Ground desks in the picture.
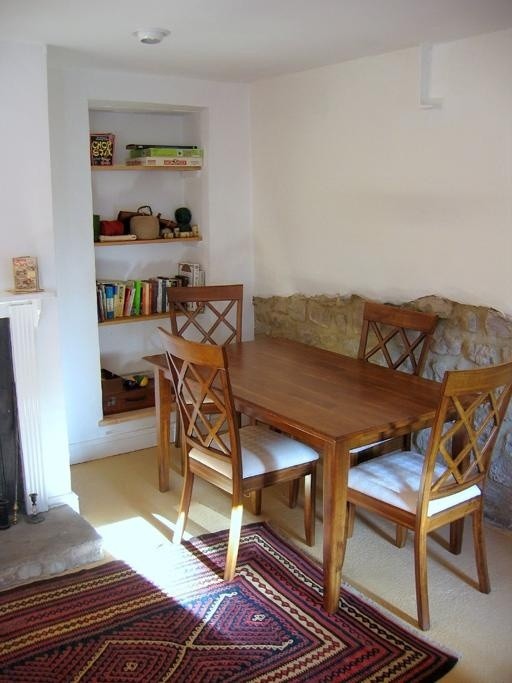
[142,335,482,616]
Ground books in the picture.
[96,263,206,323]
[98,234,137,243]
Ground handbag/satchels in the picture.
[118,205,176,229]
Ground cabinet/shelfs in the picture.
[95,166,202,427]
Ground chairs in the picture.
[344,361,511,630]
[356,302,439,462]
[159,327,319,584]
[167,286,243,448]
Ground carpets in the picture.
[2,521,459,681]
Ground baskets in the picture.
[103,377,169,416]
